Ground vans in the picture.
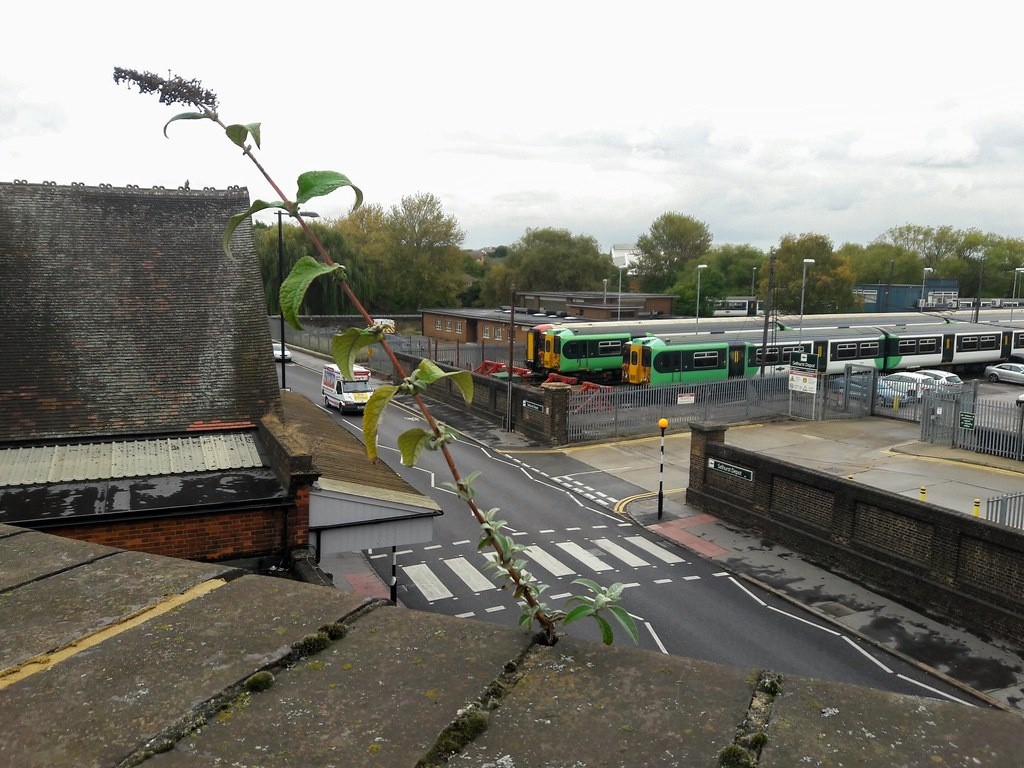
[321,364,374,415]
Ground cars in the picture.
[273,343,292,364]
[831,369,964,409]
[985,363,1024,386]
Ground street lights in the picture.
[695,264,708,336]
[920,267,933,312]
[1010,268,1024,324]
[273,209,321,388]
[603,279,608,305]
[798,258,816,353]
[618,266,623,321]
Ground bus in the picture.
[523,307,1024,403]
